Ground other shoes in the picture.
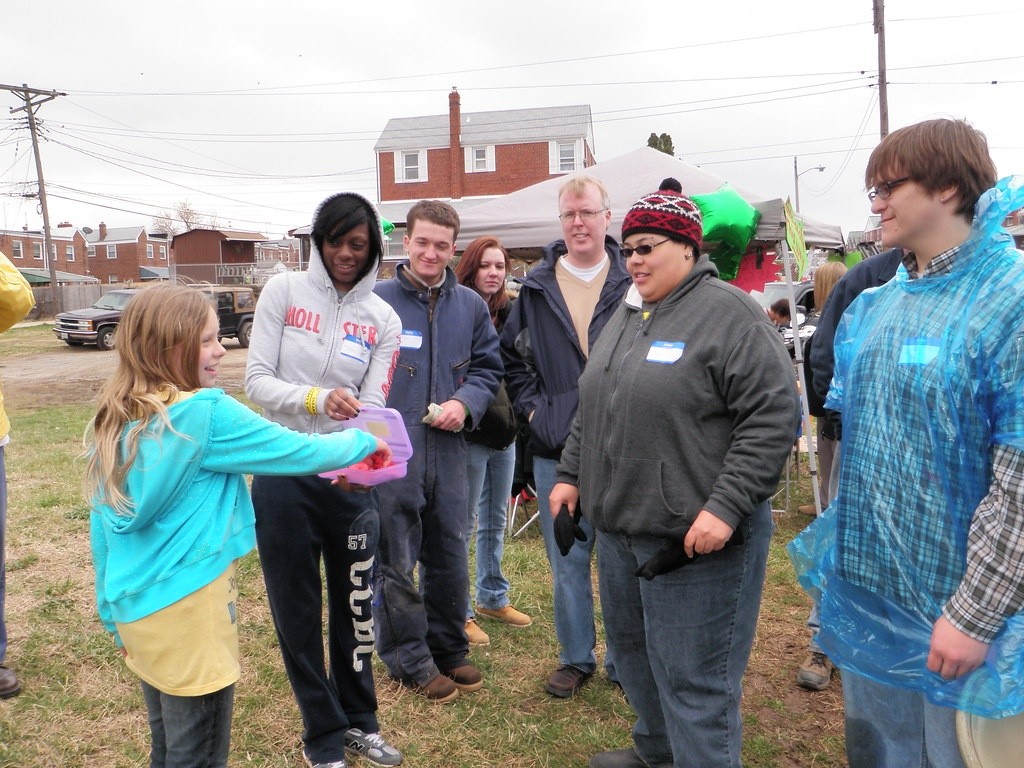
[798,505,817,514]
[1,662,22,697]
[442,665,484,691]
[590,749,645,767]
[406,673,459,702]
[545,665,588,697]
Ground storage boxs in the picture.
[315,412,419,486]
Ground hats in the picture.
[621,178,705,257]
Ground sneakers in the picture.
[463,617,491,647]
[475,604,532,628]
[302,748,347,768]
[344,727,403,767]
[797,651,835,689]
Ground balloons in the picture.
[690,180,762,280]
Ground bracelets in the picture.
[463,403,469,416]
[305,387,322,415]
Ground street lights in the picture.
[792,155,827,214]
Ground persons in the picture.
[0,251,36,699]
[786,118,1024,768]
[82,283,392,768]
[499,175,632,698]
[795,246,910,691]
[550,178,804,767]
[798,261,847,515]
[454,237,533,645]
[763,298,791,333]
[372,200,503,703]
[242,191,403,768]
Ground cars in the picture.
[50,288,146,351]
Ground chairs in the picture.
[770,396,803,513]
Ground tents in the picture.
[287,146,844,519]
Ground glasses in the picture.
[558,209,608,222]
[867,176,920,204]
[619,237,673,258]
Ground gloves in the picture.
[554,494,587,557]
[634,523,744,581]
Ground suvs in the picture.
[194,286,259,348]
